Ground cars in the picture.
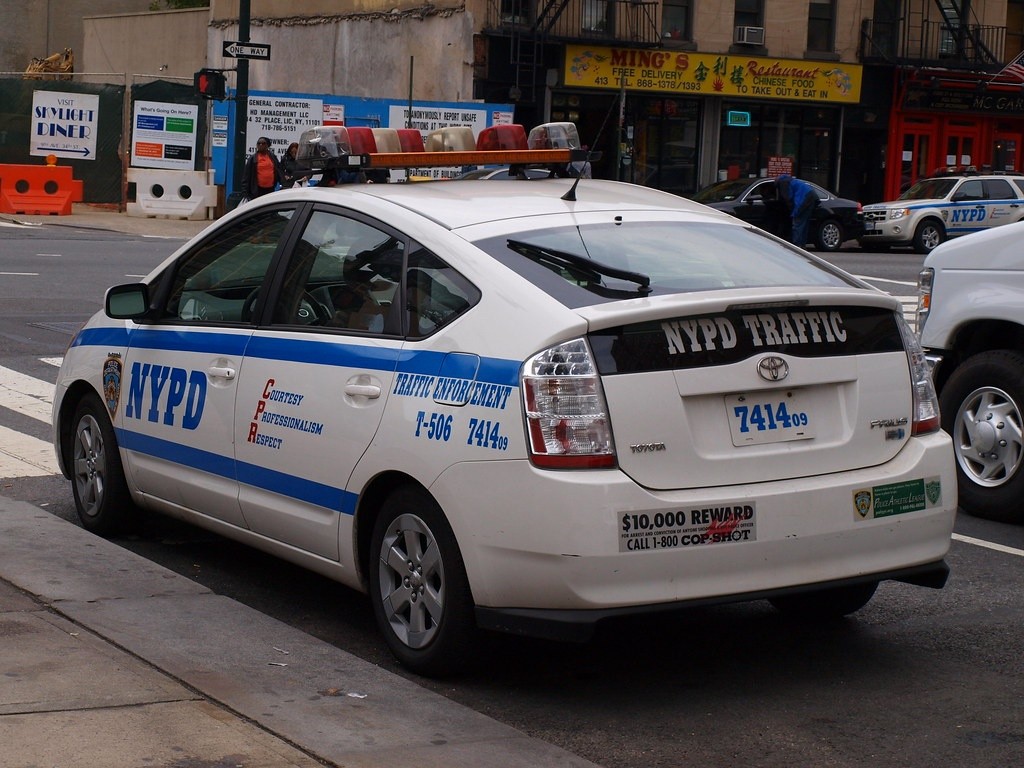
[52,121,960,678]
[691,175,865,251]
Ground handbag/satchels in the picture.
[568,162,582,179]
[236,198,248,208]
[292,181,301,189]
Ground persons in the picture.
[566,142,591,179]
[280,143,313,190]
[774,173,819,250]
[753,185,768,199]
[242,137,286,200]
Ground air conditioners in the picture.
[733,26,765,46]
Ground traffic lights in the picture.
[195,71,228,102]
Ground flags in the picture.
[1003,54,1024,81]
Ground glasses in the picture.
[257,142,267,145]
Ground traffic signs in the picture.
[221,39,272,60]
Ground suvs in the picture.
[916,218,1024,519]
[863,163,1024,254]
